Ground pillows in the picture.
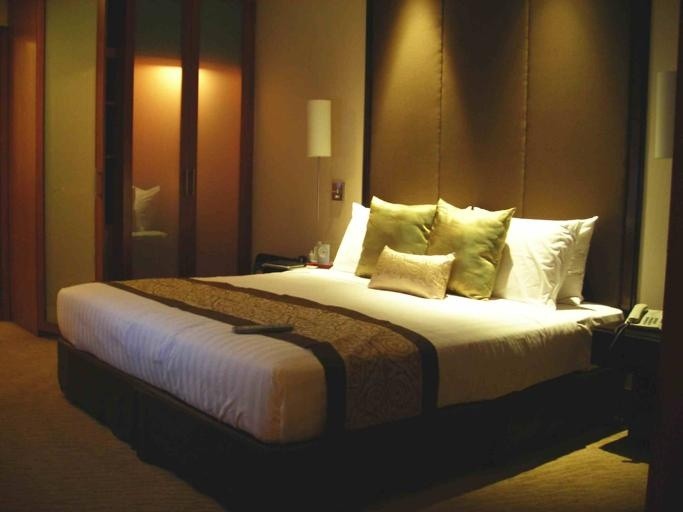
[332,195,601,304]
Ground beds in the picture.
[55,260,626,510]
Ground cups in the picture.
[318,240,330,265]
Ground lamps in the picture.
[307,99,332,252]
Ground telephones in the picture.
[625,304,663,330]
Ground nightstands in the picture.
[591,320,660,444]
[252,253,312,273]
